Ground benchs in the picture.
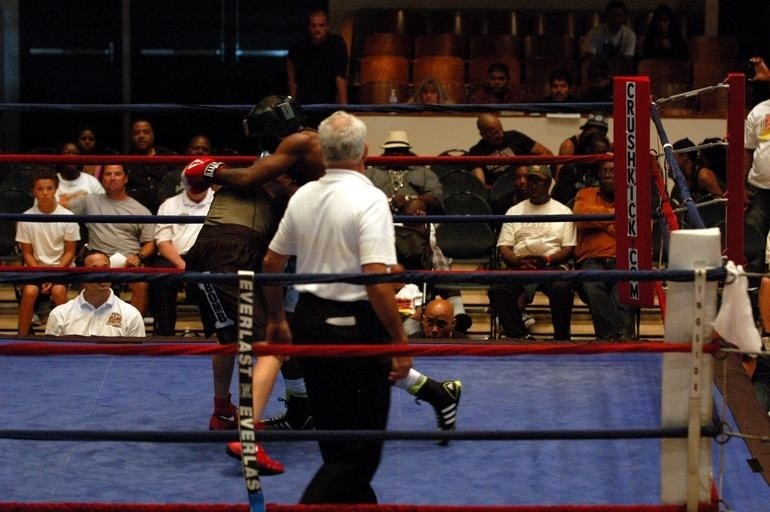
[1,134,733,344]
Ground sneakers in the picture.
[259,408,317,432]
[31,308,51,325]
[223,440,286,474]
[428,379,463,448]
[587,328,641,345]
[499,333,540,344]
[521,311,536,329]
[453,313,473,333]
[209,402,269,431]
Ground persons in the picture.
[13,2,770,351]
[185,175,293,476]
[182,91,462,447]
[265,109,414,504]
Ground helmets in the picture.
[242,95,308,155]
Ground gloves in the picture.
[179,158,226,195]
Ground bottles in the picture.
[389,88,399,115]
[183,326,193,337]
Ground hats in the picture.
[580,111,609,132]
[523,164,552,180]
[379,130,413,150]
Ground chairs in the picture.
[356,2,742,105]
